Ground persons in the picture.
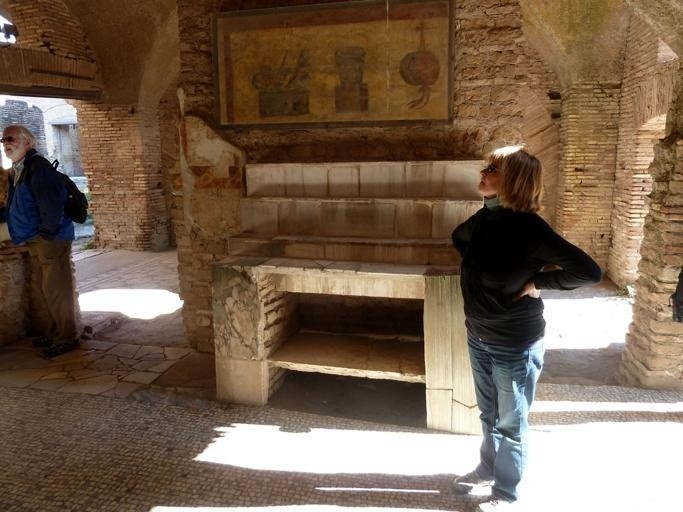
[0,123,79,360]
[452,145,604,512]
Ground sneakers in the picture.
[476,495,517,512]
[453,469,495,492]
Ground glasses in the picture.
[0,136,15,143]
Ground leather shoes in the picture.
[32,336,80,358]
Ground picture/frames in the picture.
[208,0,457,132]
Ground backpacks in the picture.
[26,155,89,224]
